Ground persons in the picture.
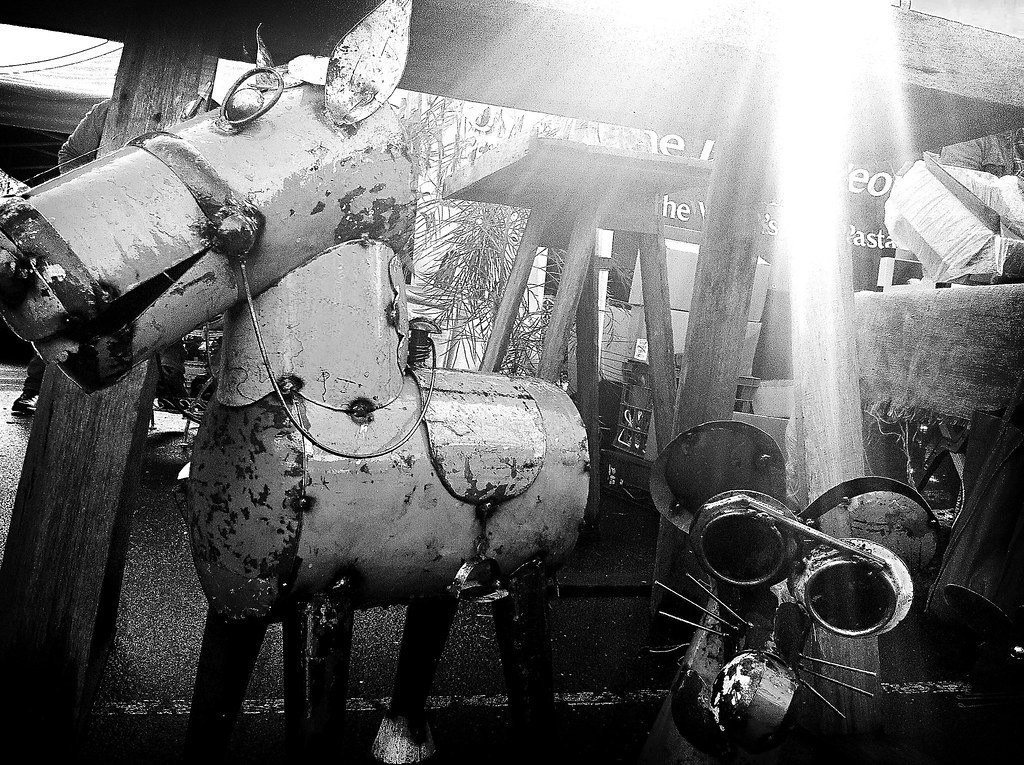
[12,101,191,414]
[941,128,1024,179]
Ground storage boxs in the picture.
[610,360,762,457]
[752,379,794,419]
[629,238,771,322]
[625,307,762,375]
